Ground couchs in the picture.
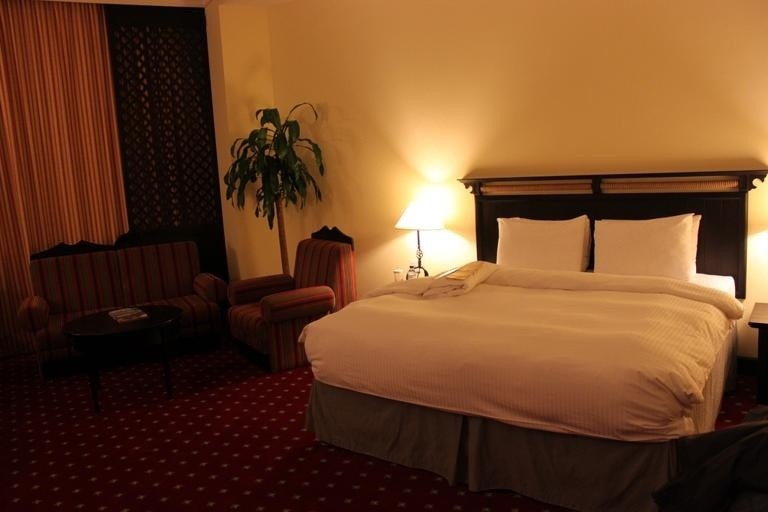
[15,224,226,385]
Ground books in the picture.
[108,305,148,325]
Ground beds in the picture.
[308,168,767,511]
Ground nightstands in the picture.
[747,303,767,407]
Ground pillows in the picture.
[493,211,703,286]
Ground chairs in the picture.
[227,226,358,374]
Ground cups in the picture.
[392,269,404,282]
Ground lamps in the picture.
[392,199,444,280]
